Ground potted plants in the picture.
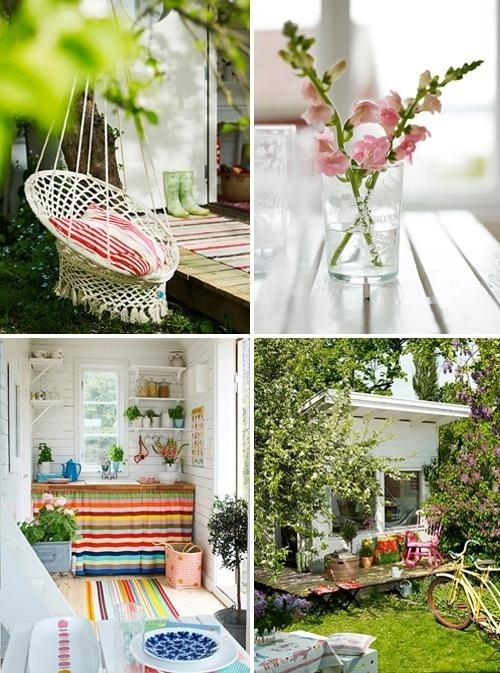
[150,437,190,472]
[108,445,123,474]
[124,406,184,429]
[37,442,53,474]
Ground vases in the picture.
[258,630,275,646]
[323,161,403,284]
[32,544,73,573]
[361,557,373,568]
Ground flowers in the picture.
[16,493,76,546]
[278,20,483,267]
[360,538,374,556]
[255,591,312,635]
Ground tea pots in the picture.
[61,458,82,481]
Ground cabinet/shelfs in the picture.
[32,355,63,428]
[128,365,188,434]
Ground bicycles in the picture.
[426,538,500,648]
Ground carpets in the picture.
[87,577,179,621]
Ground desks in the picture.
[253,630,327,672]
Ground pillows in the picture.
[51,203,168,278]
[328,632,377,656]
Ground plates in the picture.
[27,616,99,673]
[142,629,222,663]
[49,478,72,482]
[127,627,241,673]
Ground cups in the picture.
[254,123,297,285]
[133,419,142,428]
[107,603,143,672]
[31,389,60,400]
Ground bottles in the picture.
[162,409,169,427]
[137,375,181,397]
[143,415,151,427]
[139,474,155,484]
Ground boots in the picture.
[163,172,210,218]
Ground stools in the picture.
[334,579,366,611]
[307,583,339,615]
[336,648,378,673]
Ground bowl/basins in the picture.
[158,471,178,484]
[31,347,63,358]
